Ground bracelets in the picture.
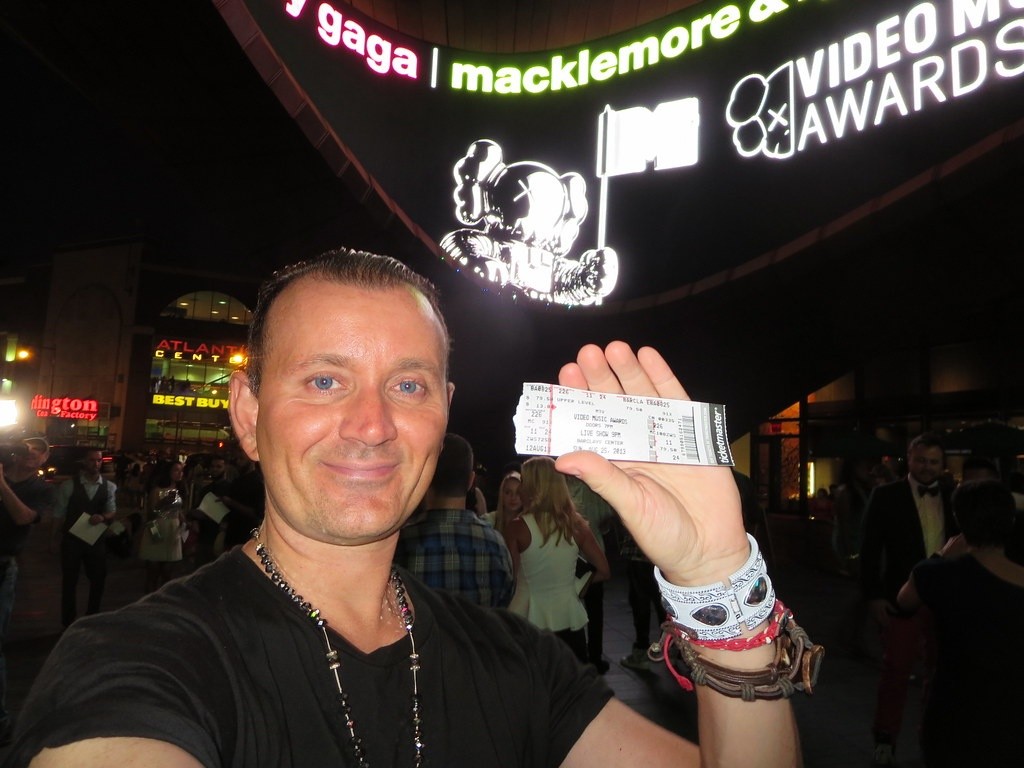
[647,532,824,702]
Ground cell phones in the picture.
[169,490,176,499]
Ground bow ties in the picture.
[918,486,940,497]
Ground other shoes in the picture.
[870,744,896,768]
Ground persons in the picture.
[0,247,823,768]
[0,437,268,744]
[391,433,684,675]
[831,431,1024,768]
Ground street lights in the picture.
[18,342,57,433]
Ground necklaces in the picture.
[250,527,426,768]
